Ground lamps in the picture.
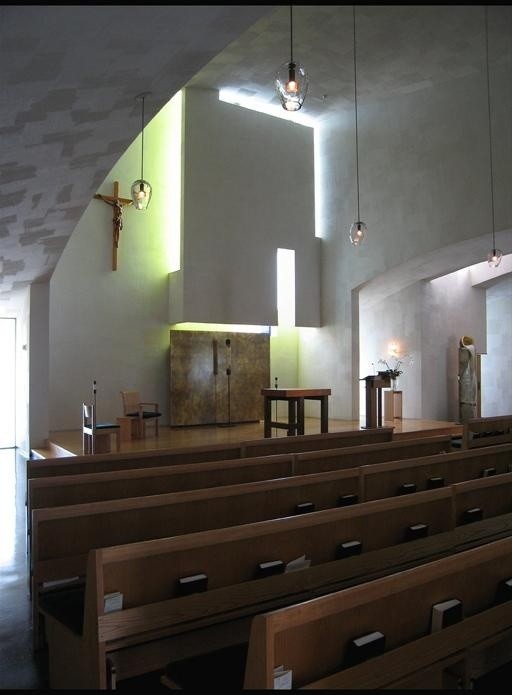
[485,3,502,272]
[347,4,370,247]
[130,85,153,213]
[273,7,308,116]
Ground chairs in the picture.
[120,390,162,439]
[83,403,122,456]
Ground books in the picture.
[405,522,430,541]
[399,483,416,496]
[464,507,485,525]
[335,494,357,507]
[483,467,496,477]
[336,540,362,558]
[255,561,285,582]
[496,578,512,607]
[343,630,386,668]
[427,478,446,490]
[431,598,464,632]
[293,502,315,515]
[177,574,209,597]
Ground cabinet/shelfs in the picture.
[169,330,271,428]
[384,391,403,422]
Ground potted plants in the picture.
[380,360,404,390]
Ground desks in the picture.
[261,388,331,439]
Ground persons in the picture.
[98,195,134,248]
